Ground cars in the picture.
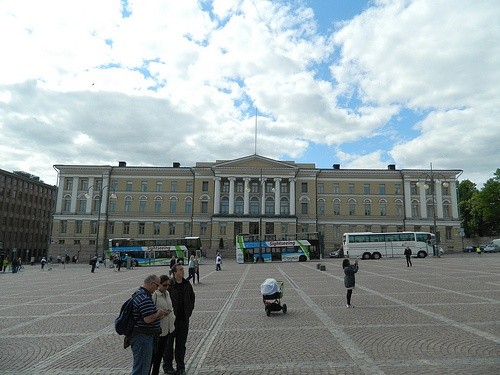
[484,244,500,253]
[466,245,485,252]
[330,248,343,258]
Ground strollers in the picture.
[260,277,288,316]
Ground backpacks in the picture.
[116,291,151,334]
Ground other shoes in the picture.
[176,366,186,375]
[163,366,177,375]
[346,304,354,308]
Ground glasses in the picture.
[161,283,170,287]
[153,281,160,288]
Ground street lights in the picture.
[85,184,117,263]
[416,162,449,258]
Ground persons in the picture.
[30,255,35,265]
[343,259,358,308]
[215,252,221,271]
[170,255,175,279]
[91,254,97,273]
[167,264,195,375]
[12,257,17,273]
[194,255,202,283]
[177,254,184,265]
[476,246,482,258]
[185,255,197,285]
[404,245,412,267]
[151,274,177,375]
[220,253,224,264]
[16,257,22,272]
[113,255,121,272]
[41,254,79,271]
[126,256,134,270]
[0,256,8,272]
[127,275,160,375]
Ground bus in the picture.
[109,237,205,267]
[235,232,325,264]
[342,231,435,260]
